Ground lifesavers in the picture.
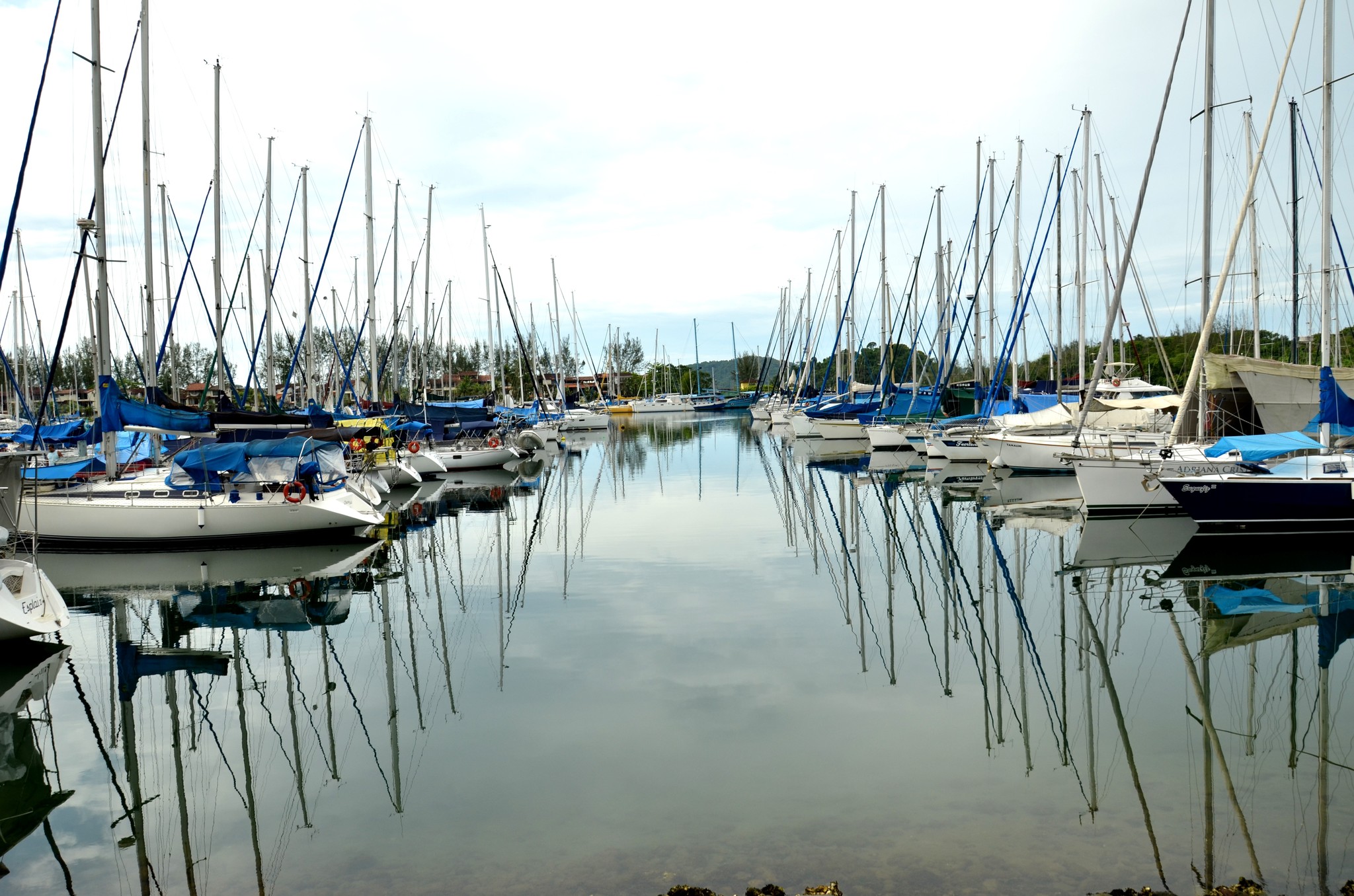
[1112,379,1120,387]
[290,579,310,600]
[284,481,306,503]
[370,435,384,446]
[349,438,364,450]
[490,488,502,499]
[412,503,423,516]
[489,437,498,448]
[408,442,421,454]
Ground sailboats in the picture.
[0,0,1354,638]
[0,413,1353,895]
